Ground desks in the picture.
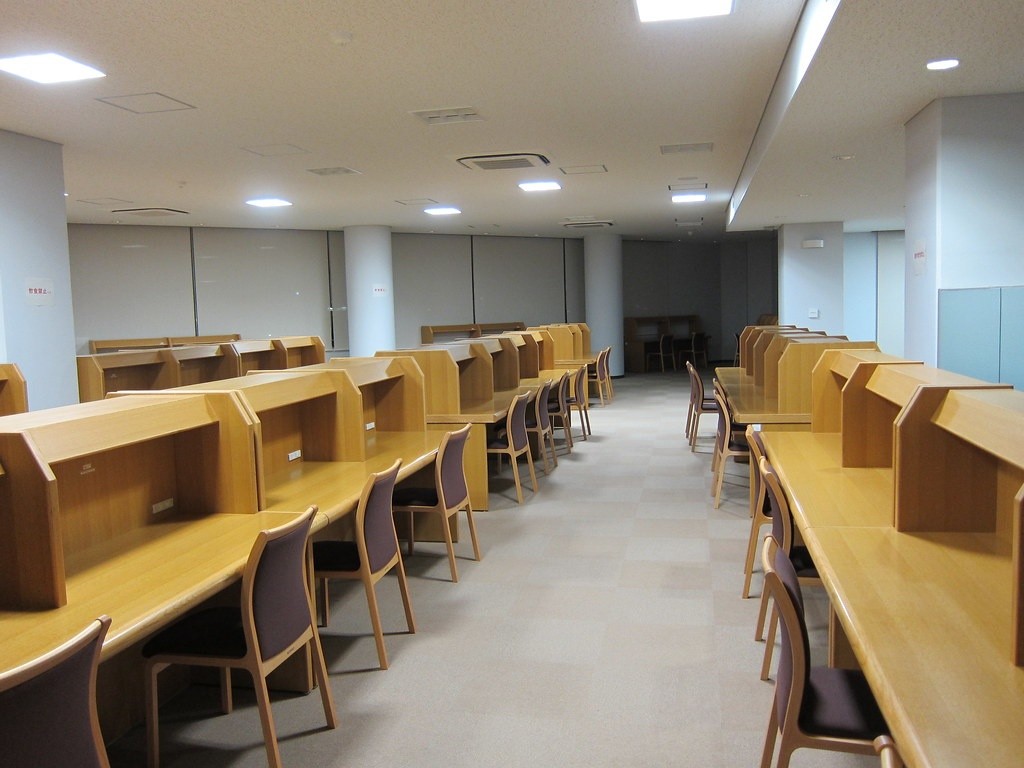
[0,314,1024,768]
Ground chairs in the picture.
[684,360,719,439]
[392,422,481,583]
[0,613,112,768]
[710,377,750,481]
[688,366,724,453]
[311,456,416,671]
[678,331,708,371]
[753,455,852,682]
[741,423,792,600]
[760,531,896,768]
[709,388,764,520]
[487,389,539,505]
[497,378,558,475]
[646,333,677,373]
[564,363,592,441]
[733,332,739,367]
[142,503,339,768]
[547,370,574,455]
[589,346,615,398]
[872,734,907,767]
[587,350,610,408]
